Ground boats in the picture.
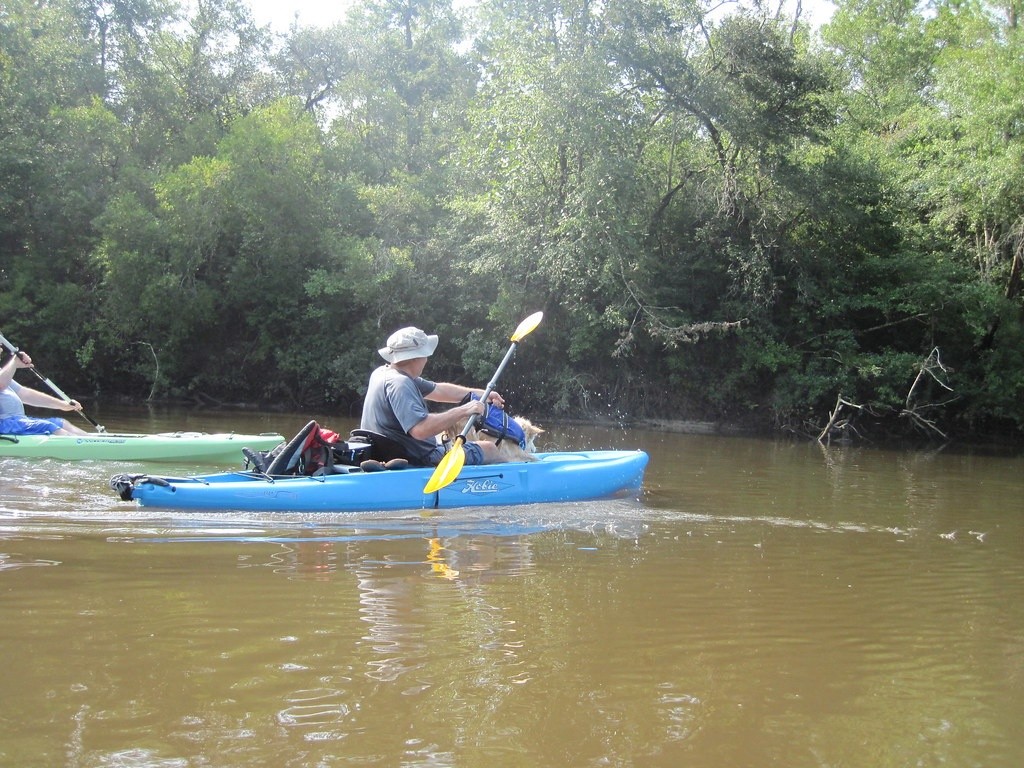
[1,429,286,467]
[116,427,654,513]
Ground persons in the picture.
[360,325,545,468]
[0,342,94,437]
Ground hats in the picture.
[378,327,438,363]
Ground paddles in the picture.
[423,310,543,494]
[0,335,107,433]
